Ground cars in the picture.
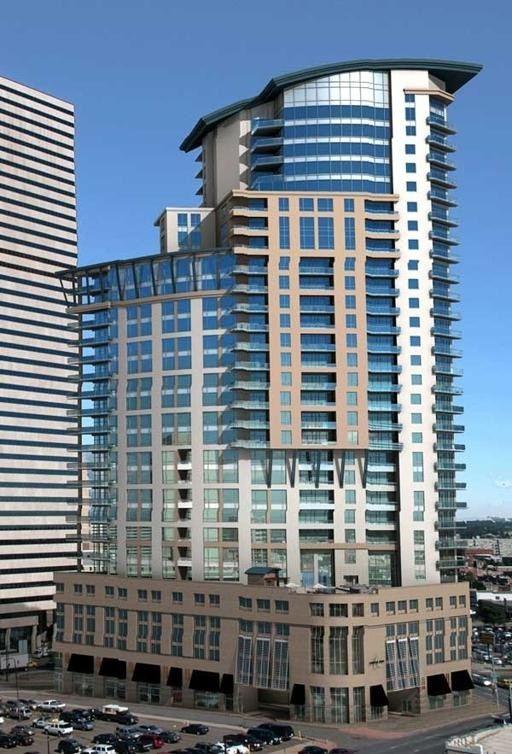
[297,745,328,754]
[35,646,50,659]
[470,626,512,690]
[329,748,350,754]
[0,698,294,754]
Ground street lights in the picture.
[3,657,20,701]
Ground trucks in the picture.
[1,653,38,675]
